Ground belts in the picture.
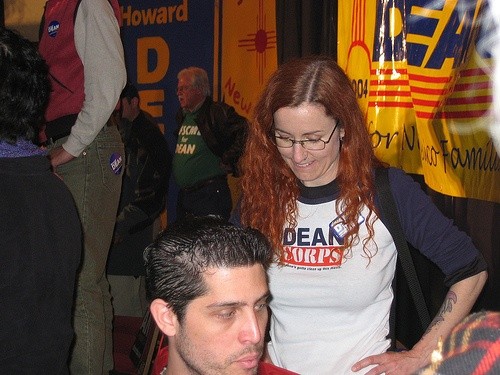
[184,175,228,193]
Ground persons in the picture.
[226,56,488,375]
[0,27,82,375]
[38,0,128,375]
[105,67,300,375]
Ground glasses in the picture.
[268,121,339,150]
